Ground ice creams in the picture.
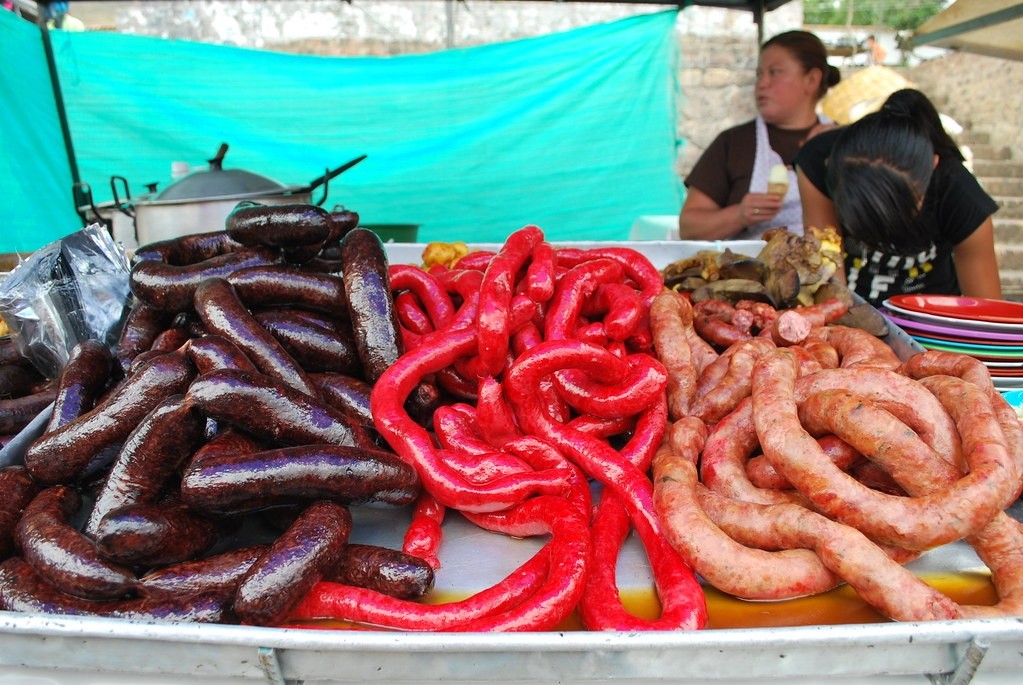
[767,164,789,199]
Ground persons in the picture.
[678,31,840,241]
[793,90,1005,309]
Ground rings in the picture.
[752,209,759,215]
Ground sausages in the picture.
[1,199,1023,632]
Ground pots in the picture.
[111,145,329,248]
[72,182,158,251]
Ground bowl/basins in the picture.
[360,224,421,244]
[880,295,1023,392]
